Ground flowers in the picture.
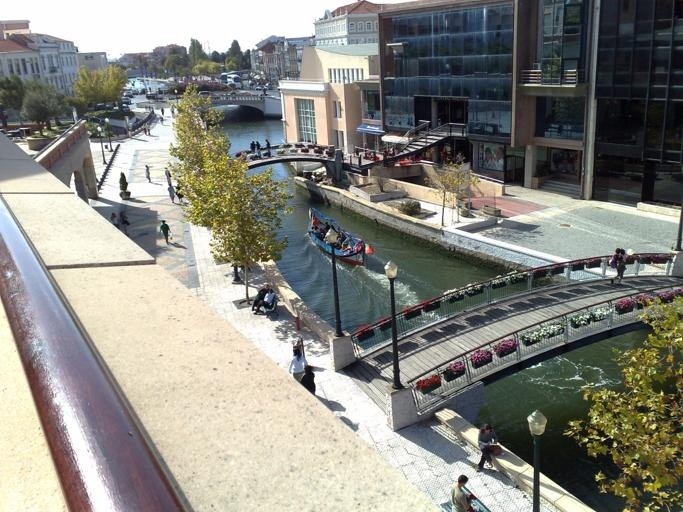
[411,330,542,395]
[349,252,676,341]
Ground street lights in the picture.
[104,117,114,153]
[323,226,345,340]
[525,409,548,511]
[383,258,405,392]
[174,88,180,105]
[96,125,107,164]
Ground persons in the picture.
[110,212,118,230]
[616,248,628,270]
[492,146,504,171]
[174,183,184,203]
[142,121,146,136]
[482,147,494,169]
[449,474,476,512]
[289,347,308,385]
[611,247,626,286]
[294,335,304,358]
[382,147,388,160]
[251,284,272,312]
[163,167,171,188]
[167,182,174,204]
[253,288,276,316]
[475,422,498,474]
[159,219,170,246]
[300,372,314,396]
[158,115,163,125]
[249,141,255,153]
[144,165,151,182]
[264,139,271,157]
[118,211,128,235]
[365,145,373,160]
[310,212,355,252]
[160,106,163,116]
[170,106,174,118]
[146,122,150,136]
[255,141,261,158]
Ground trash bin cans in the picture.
[292,340,301,356]
[104,145,108,149]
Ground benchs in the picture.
[258,300,279,318]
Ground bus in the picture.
[218,68,267,92]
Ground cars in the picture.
[197,90,220,100]
[255,84,274,91]
[94,78,164,109]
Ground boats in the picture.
[307,206,367,266]
